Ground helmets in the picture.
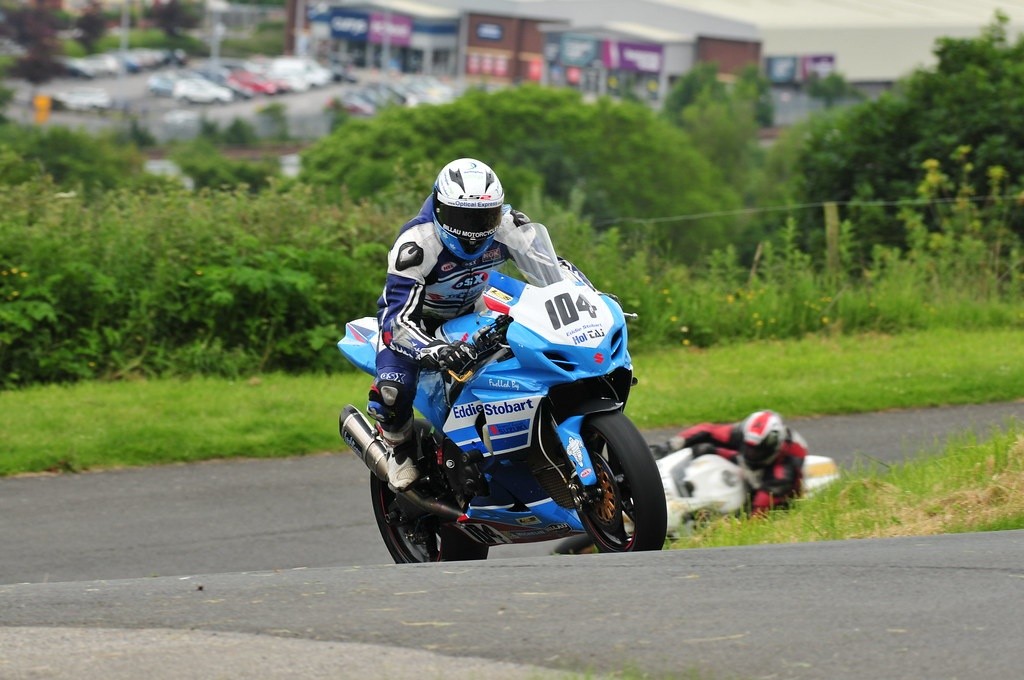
[433,158,504,261]
[741,410,784,468]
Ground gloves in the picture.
[420,339,477,373]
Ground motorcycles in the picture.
[553,439,840,555]
[335,222,669,568]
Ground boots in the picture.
[376,407,422,493]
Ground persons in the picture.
[650,409,808,520]
[368,157,565,492]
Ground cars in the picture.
[35,48,450,121]
